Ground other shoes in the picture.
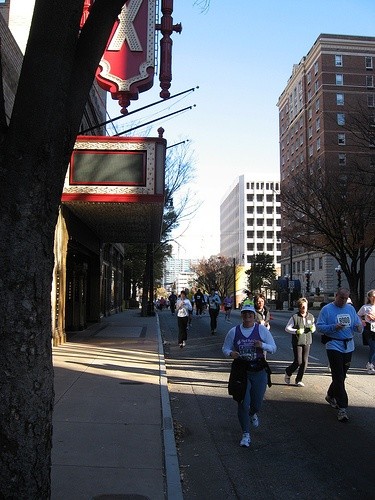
[212,329,216,334]
[180,341,186,349]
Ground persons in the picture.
[317,287,363,422]
[156,287,375,391]
[222,306,278,448]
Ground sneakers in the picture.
[366,362,375,374]
[295,381,305,387]
[240,435,251,447]
[325,395,340,408]
[250,413,258,426]
[285,371,290,384]
[337,407,349,420]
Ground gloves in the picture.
[310,326,314,331]
[297,329,304,335]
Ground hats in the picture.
[241,305,255,314]
